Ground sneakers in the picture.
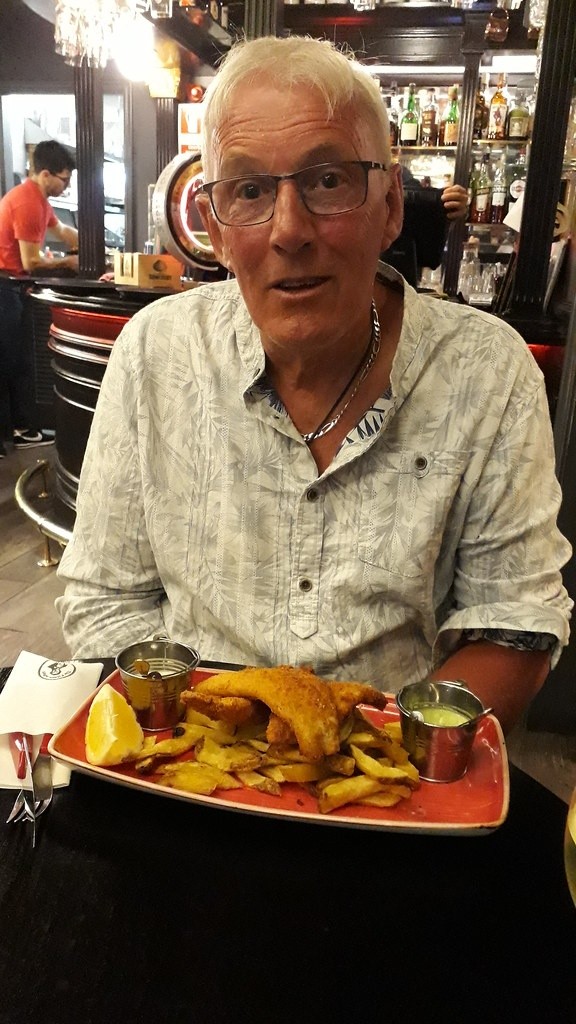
[13,428,55,450]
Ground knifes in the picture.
[8,732,36,847]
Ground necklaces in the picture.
[304,303,375,448]
[296,296,380,441]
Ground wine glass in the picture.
[562,96,576,157]
[458,259,508,297]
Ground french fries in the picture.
[121,703,421,814]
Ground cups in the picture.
[418,264,445,295]
[114,635,202,733]
[395,681,485,784]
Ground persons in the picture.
[51,34,575,742]
[380,117,469,288]
[2,139,85,457]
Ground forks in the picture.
[5,733,55,824]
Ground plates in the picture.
[46,668,511,836]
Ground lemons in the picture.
[84,682,143,765]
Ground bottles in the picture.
[420,88,437,147]
[470,76,488,140]
[506,146,529,221]
[507,94,531,140]
[464,152,492,223]
[400,81,420,146]
[386,81,399,146]
[439,83,463,146]
[491,150,511,224]
[485,72,510,140]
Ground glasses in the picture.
[51,173,70,189]
[200,157,389,227]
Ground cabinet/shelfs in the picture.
[167,70,572,331]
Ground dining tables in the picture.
[2,660,575,1022]
